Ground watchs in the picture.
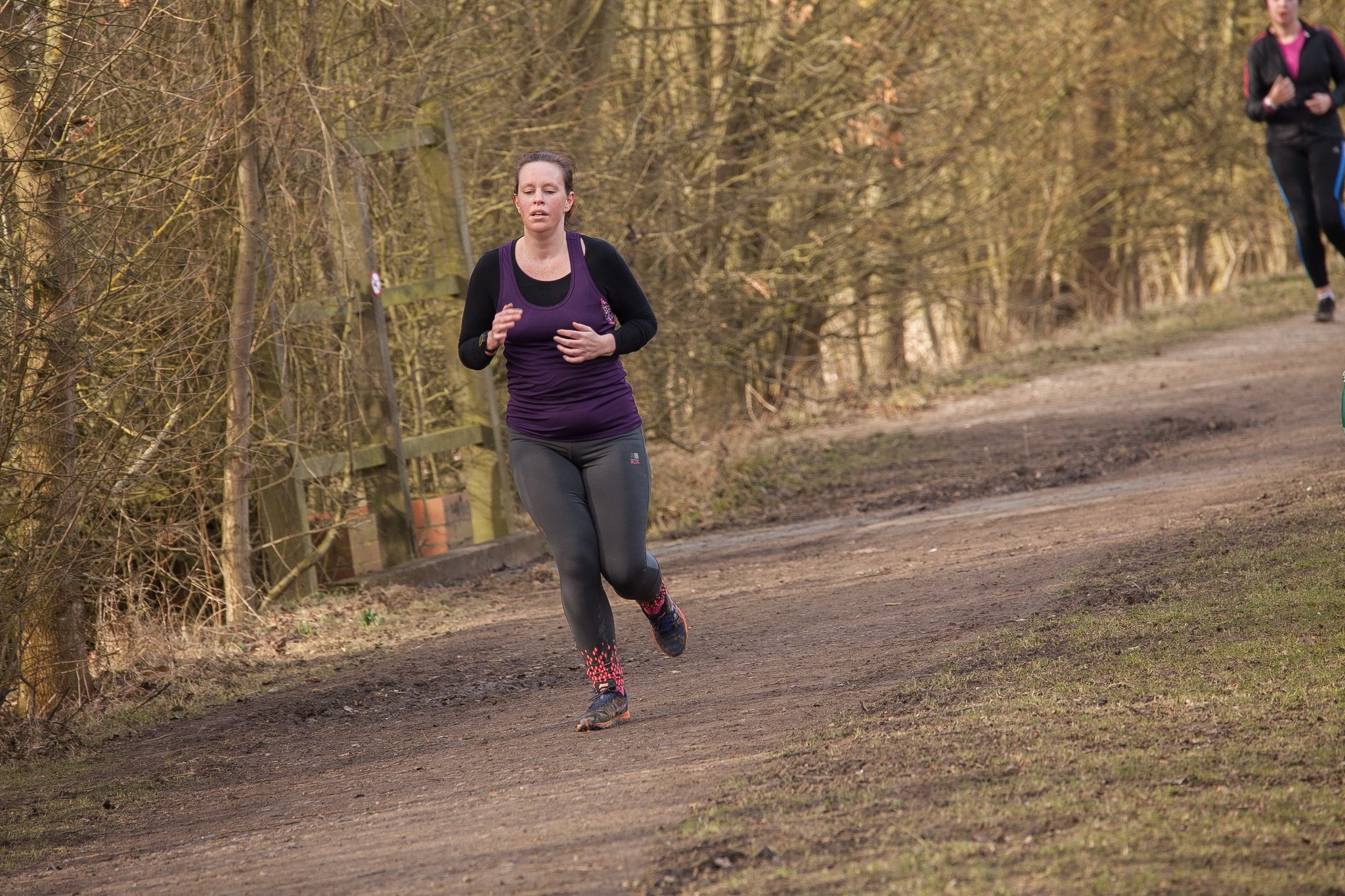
[1264,96,1277,109]
[479,331,500,354]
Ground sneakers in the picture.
[1315,297,1336,321]
[637,578,687,658]
[577,683,630,732]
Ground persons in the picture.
[457,151,689,733]
[1242,0,1345,323]
